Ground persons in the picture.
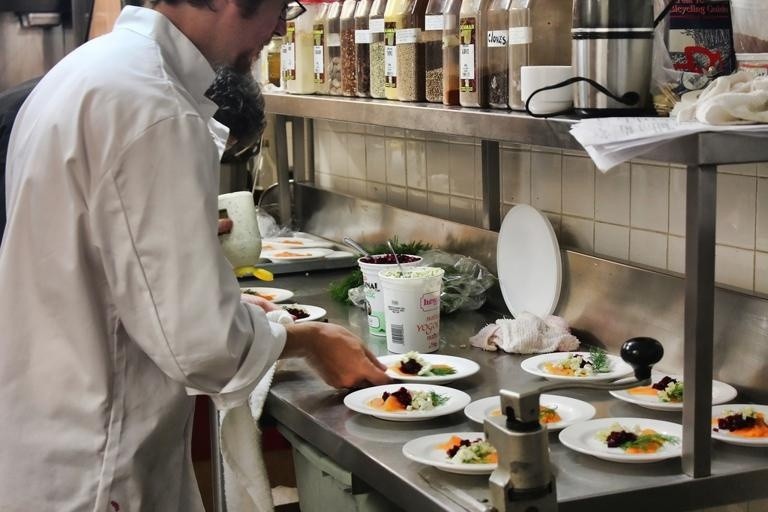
[2,58,264,248]
[2,1,393,512]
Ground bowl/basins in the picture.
[520,64,576,114]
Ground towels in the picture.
[471,315,579,355]
[218,311,297,511]
[695,70,767,125]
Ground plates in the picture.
[609,371,735,413]
[495,203,561,319]
[239,284,292,304]
[262,237,316,249]
[465,392,596,431]
[398,432,551,474]
[342,384,469,424]
[260,249,334,263]
[325,250,353,259]
[373,353,481,381]
[709,405,768,447]
[518,349,634,383]
[273,303,328,323]
[554,415,683,463]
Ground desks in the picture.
[191,264,768,512]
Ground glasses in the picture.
[279,2,308,22]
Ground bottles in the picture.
[250,139,278,203]
[267,37,282,86]
[282,1,571,112]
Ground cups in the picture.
[355,254,422,337]
[377,265,446,353]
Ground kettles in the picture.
[568,1,656,115]
